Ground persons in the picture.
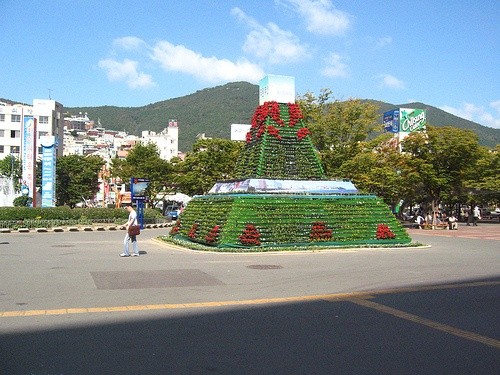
[177,205,184,218]
[401,206,481,230]
[119,204,140,257]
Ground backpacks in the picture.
[420,218,423,224]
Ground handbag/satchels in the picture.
[479,214,481,219]
[129,226,140,236]
[428,220,432,224]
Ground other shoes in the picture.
[131,251,139,257]
[120,252,131,257]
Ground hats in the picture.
[474,206,479,209]
